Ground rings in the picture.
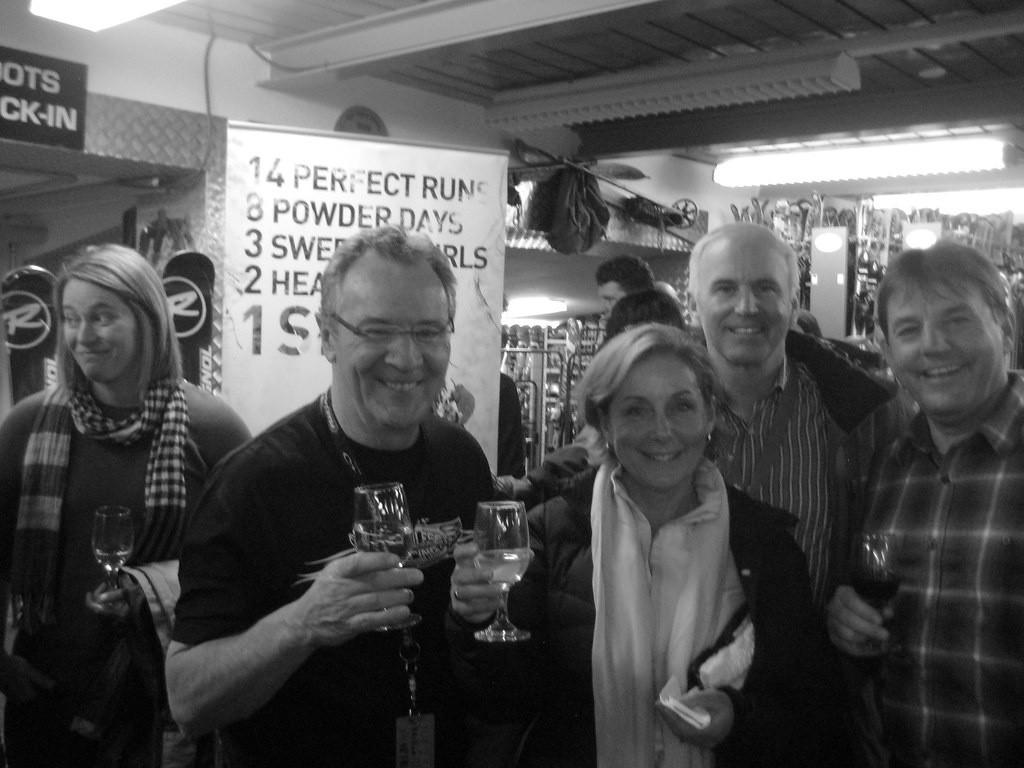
[454,589,459,599]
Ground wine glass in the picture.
[854,527,903,656]
[91,506,134,608]
[474,502,531,642]
[352,483,422,631]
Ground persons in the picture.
[825,243,1024,768]
[166,228,503,768]
[0,244,253,768]
[452,222,898,768]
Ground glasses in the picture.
[321,298,455,350]
